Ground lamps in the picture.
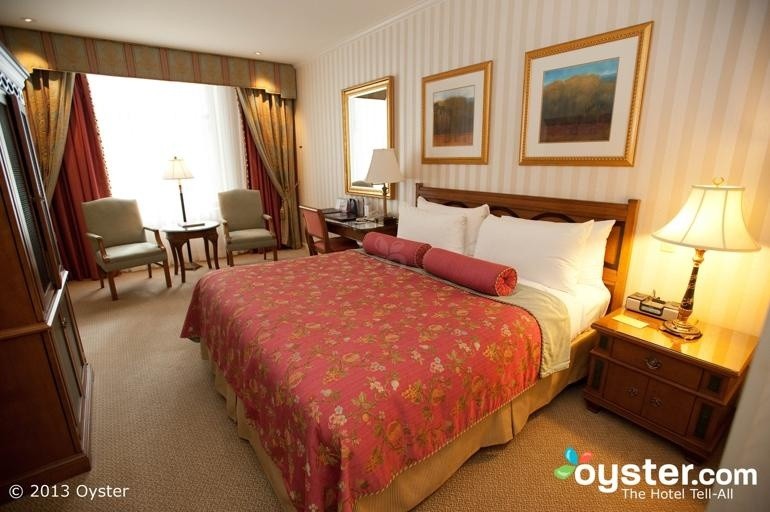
[161,157,201,270]
[364,148,403,217]
[650,184,762,341]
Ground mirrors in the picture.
[341,76,395,199]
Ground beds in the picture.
[180,183,639,512]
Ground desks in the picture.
[325,210,398,243]
[161,220,220,282]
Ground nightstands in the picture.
[582,306,760,465]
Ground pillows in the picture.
[416,196,489,257]
[502,215,616,295]
[422,247,517,297]
[363,230,431,268]
[473,213,595,294]
[398,200,467,253]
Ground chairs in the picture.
[299,206,360,255]
[81,198,172,300]
[217,189,278,267]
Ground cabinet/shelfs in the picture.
[0,42,95,507]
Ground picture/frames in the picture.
[421,60,492,165]
[517,22,652,165]
